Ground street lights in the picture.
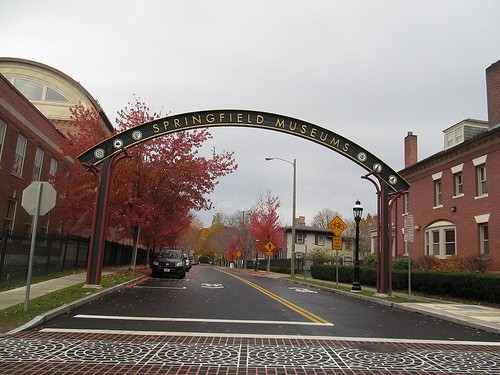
[265,156,297,279]
[352,199,363,291]
[240,209,245,269]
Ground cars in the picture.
[150,248,187,279]
[182,253,194,272]
